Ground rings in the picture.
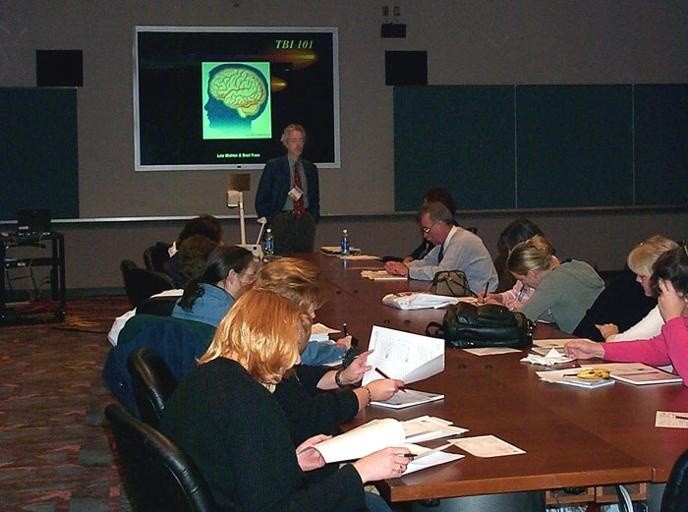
[398,465,403,471]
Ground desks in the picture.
[252,245,688,512]
[0,230,65,323]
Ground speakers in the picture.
[17,208,51,234]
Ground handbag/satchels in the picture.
[429,271,476,296]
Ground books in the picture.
[301,414,466,484]
[583,360,683,389]
[397,414,459,444]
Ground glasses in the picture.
[232,268,256,284]
[286,137,303,143]
[420,220,444,232]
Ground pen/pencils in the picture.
[676,416,688,420]
[375,367,407,393]
[397,453,417,457]
[482,281,489,303]
[511,284,525,310]
[342,323,348,337]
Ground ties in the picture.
[293,162,304,216]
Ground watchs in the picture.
[334,366,345,390]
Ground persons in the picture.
[594,234,679,372]
[106,235,219,345]
[234,257,322,357]
[505,233,606,334]
[477,216,559,310]
[380,188,460,262]
[563,235,688,388]
[254,123,321,252]
[383,201,499,296]
[170,242,353,364]
[152,288,415,512]
[146,213,225,274]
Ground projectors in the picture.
[227,189,267,258]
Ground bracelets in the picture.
[359,385,371,408]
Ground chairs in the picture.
[126,346,179,422]
[104,402,218,512]
[135,300,175,318]
[120,258,136,276]
[465,228,477,233]
[122,268,178,309]
[661,446,687,512]
[143,245,166,269]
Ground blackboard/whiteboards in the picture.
[393,84,687,211]
[7,87,80,220]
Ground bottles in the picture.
[341,228,350,255]
[264,229,274,256]
